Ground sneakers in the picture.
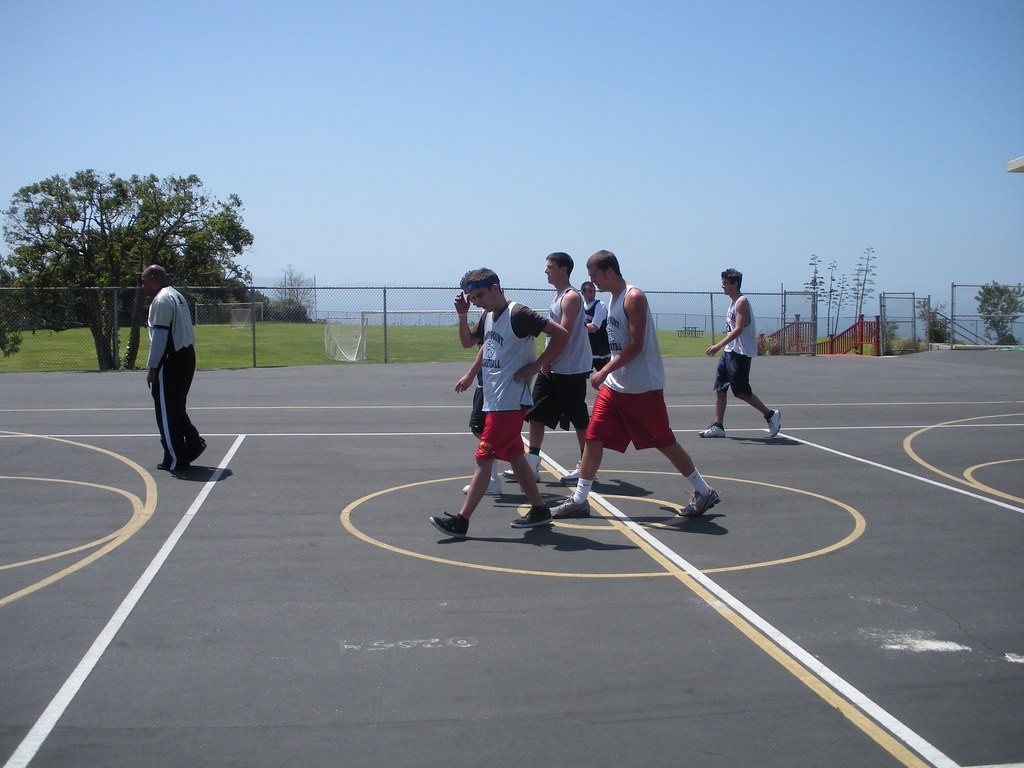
[549,496,590,518]
[463,477,502,495]
[679,487,720,517]
[561,460,599,484]
[510,503,552,527]
[526,452,542,473]
[767,409,781,437]
[503,469,540,482]
[430,511,468,538]
[699,424,725,437]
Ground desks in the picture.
[682,327,699,338]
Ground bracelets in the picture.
[458,313,467,316]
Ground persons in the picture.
[503,252,599,483]
[143,265,207,471]
[698,268,782,437]
[550,250,722,516]
[454,268,542,493]
[581,282,610,379]
[429,268,569,538]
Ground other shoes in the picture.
[189,443,206,462]
[157,463,169,469]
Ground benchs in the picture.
[677,329,704,337]
